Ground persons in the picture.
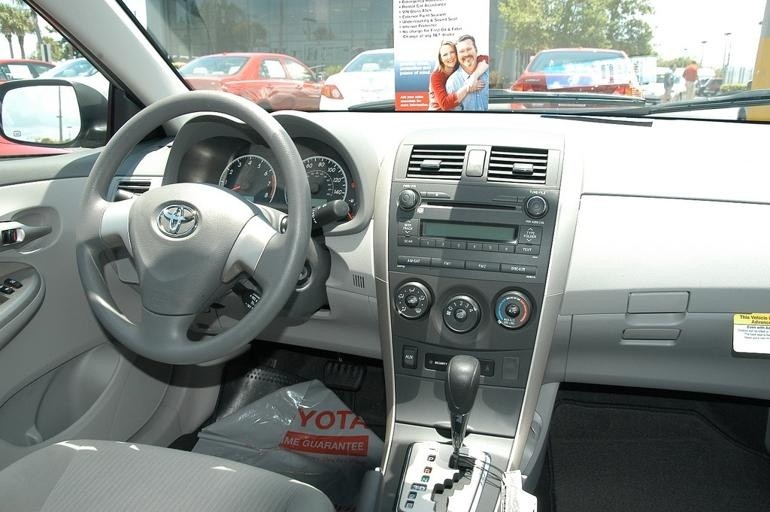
[442,34,490,111]
[663,62,677,101]
[427,40,490,113]
[681,60,699,100]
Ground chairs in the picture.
[0,439,336,512]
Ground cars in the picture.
[511,48,644,110]
[694,77,725,95]
